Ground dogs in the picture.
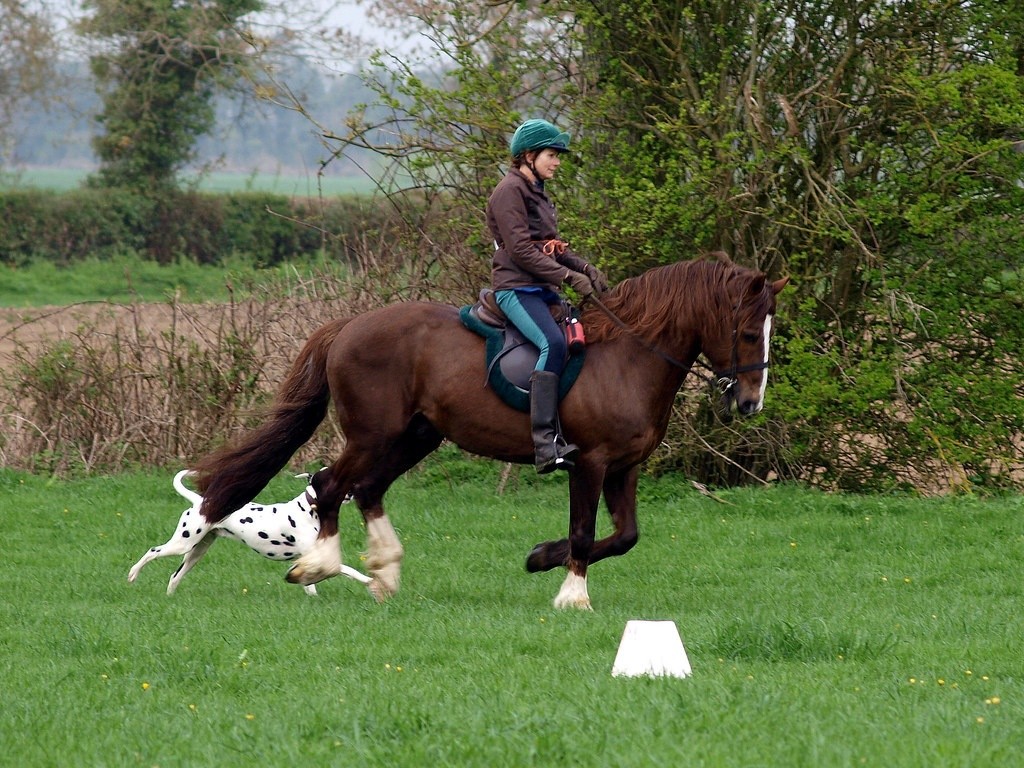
[127,466,374,596]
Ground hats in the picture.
[510,118,571,158]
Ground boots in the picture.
[530,370,581,474]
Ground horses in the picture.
[187,251,792,611]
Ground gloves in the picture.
[583,263,608,294]
[563,269,593,297]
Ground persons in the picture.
[486,119,609,474]
